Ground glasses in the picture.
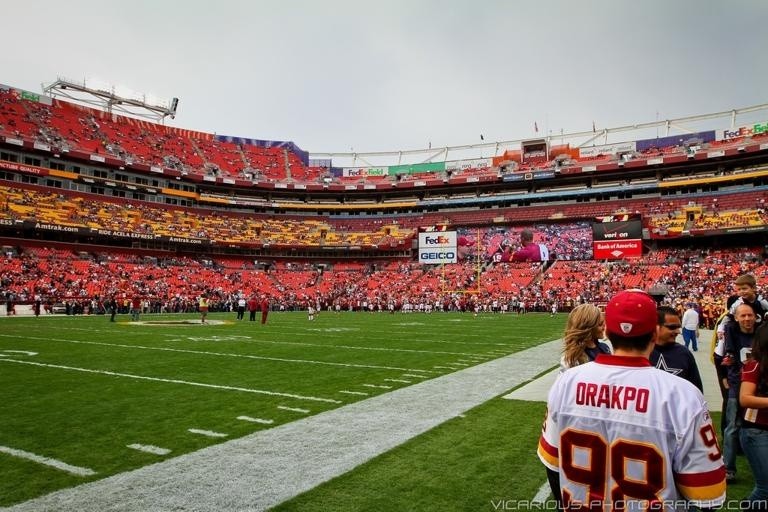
[664,323,682,329]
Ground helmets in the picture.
[604,289,656,337]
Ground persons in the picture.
[536,288,727,512]
[649,306,704,395]
[736,316,768,512]
[1,87,768,511]
[557,303,612,375]
[713,304,768,485]
[681,303,700,351]
